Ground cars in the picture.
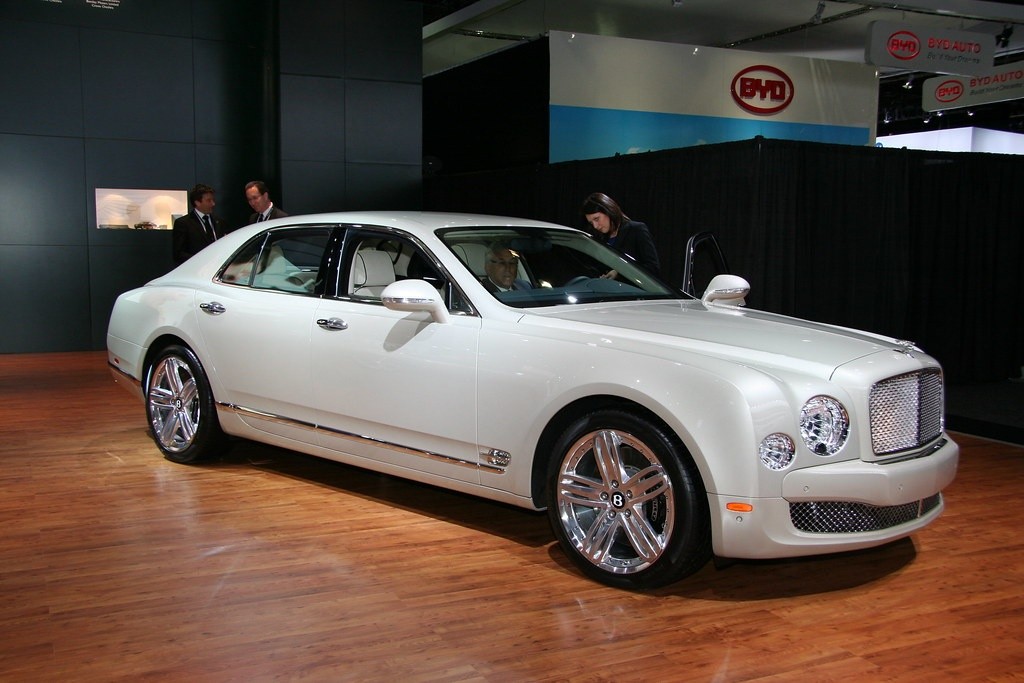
[107,211,962,592]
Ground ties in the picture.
[258,213,264,222]
[202,215,215,243]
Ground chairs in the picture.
[451,244,488,280]
[352,251,396,297]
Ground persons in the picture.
[481,242,536,292]
[171,183,237,267]
[584,192,672,286]
[244,180,288,224]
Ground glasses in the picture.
[489,259,519,265]
[247,194,263,200]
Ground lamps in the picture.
[809,2,825,25]
